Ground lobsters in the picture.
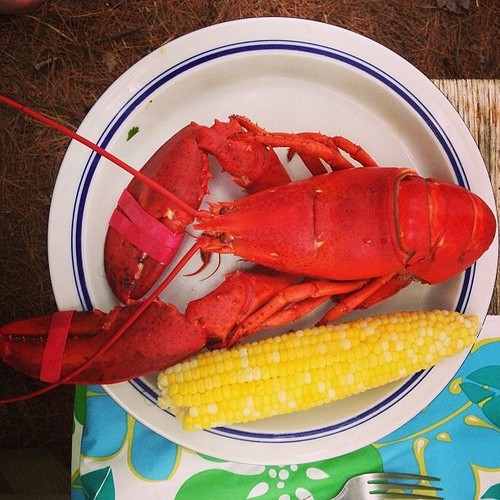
[0,96,497,406]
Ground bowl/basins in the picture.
[47,17,499,465]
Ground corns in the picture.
[154,308,482,432]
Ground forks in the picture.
[329,472,445,500]
